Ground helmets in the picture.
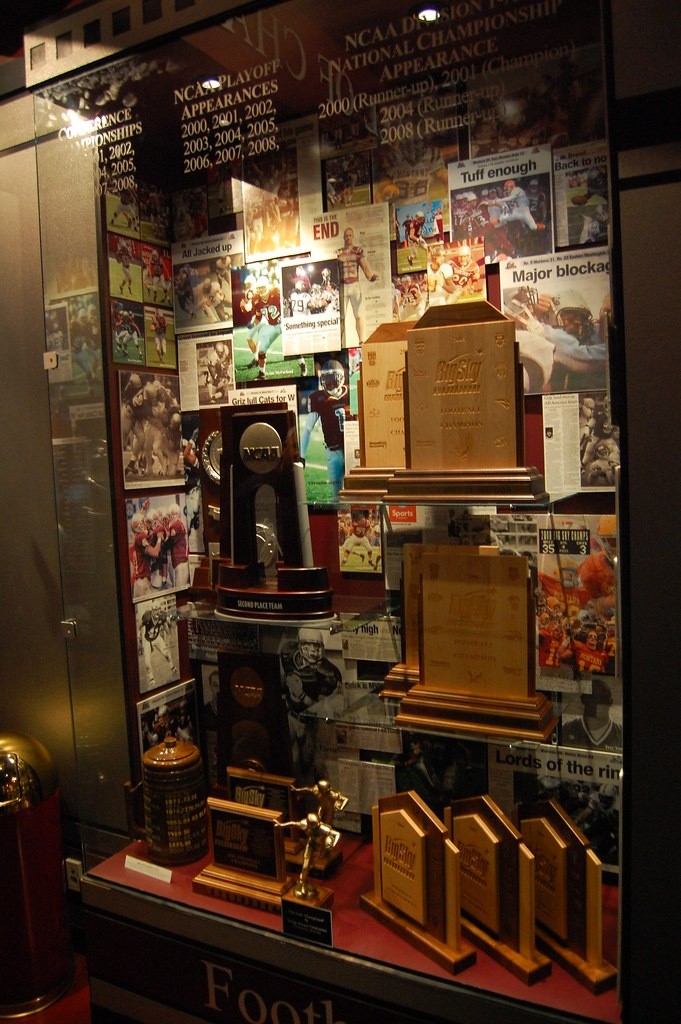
[298,628,325,663]
[456,179,539,203]
[430,246,471,265]
[598,784,617,815]
[120,241,159,261]
[415,211,426,223]
[243,273,270,298]
[128,373,182,424]
[294,280,307,293]
[131,503,182,533]
[150,601,161,610]
[547,290,593,327]
[596,516,617,539]
[78,303,97,325]
[318,360,345,394]
[538,595,615,633]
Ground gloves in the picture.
[589,796,599,810]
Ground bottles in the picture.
[142,737,209,865]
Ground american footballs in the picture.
[571,195,588,205]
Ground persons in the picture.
[284,265,331,343]
[579,398,621,486]
[216,161,233,212]
[108,196,202,484]
[140,600,179,687]
[248,170,300,251]
[573,784,619,867]
[272,812,341,897]
[507,284,608,391]
[289,777,349,823]
[280,625,343,775]
[59,304,102,401]
[305,359,349,501]
[238,274,307,378]
[333,226,378,347]
[129,502,190,590]
[538,514,620,670]
[338,508,381,571]
[146,705,194,743]
[319,72,608,324]
[555,676,622,751]
[206,341,232,404]
[175,253,238,325]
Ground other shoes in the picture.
[118,284,171,305]
[251,370,265,381]
[124,457,182,479]
[116,344,143,360]
[246,359,260,369]
[299,363,307,376]
[406,256,414,267]
